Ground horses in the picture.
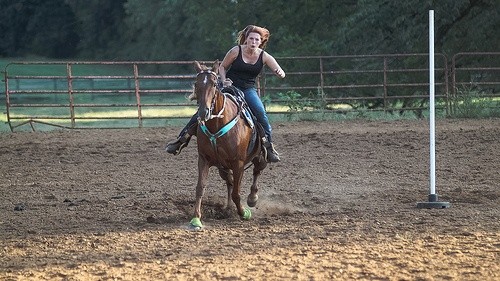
[188,59,268,228]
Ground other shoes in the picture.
[266,147,280,162]
[166,140,185,154]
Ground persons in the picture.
[165,25,285,162]
